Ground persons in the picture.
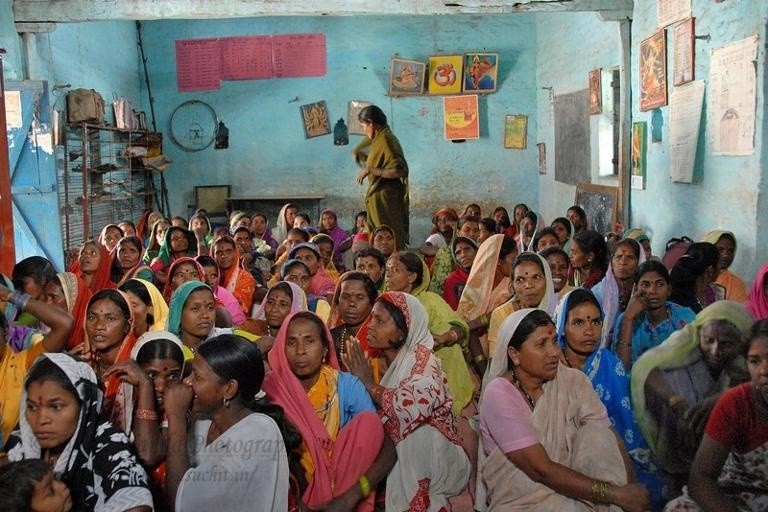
[353,106,409,251]
[1,202,768,511]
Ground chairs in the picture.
[187,185,230,233]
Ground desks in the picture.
[225,195,325,228]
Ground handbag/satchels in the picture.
[113,98,147,131]
[67,89,105,124]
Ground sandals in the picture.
[72,163,134,205]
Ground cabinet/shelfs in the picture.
[61,123,151,270]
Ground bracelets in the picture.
[379,169,384,178]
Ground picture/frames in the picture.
[389,58,425,97]
[673,16,695,86]
[631,122,647,191]
[462,53,499,94]
[348,101,372,136]
[639,29,668,112]
[300,102,330,139]
[504,115,528,150]
[428,56,464,95]
